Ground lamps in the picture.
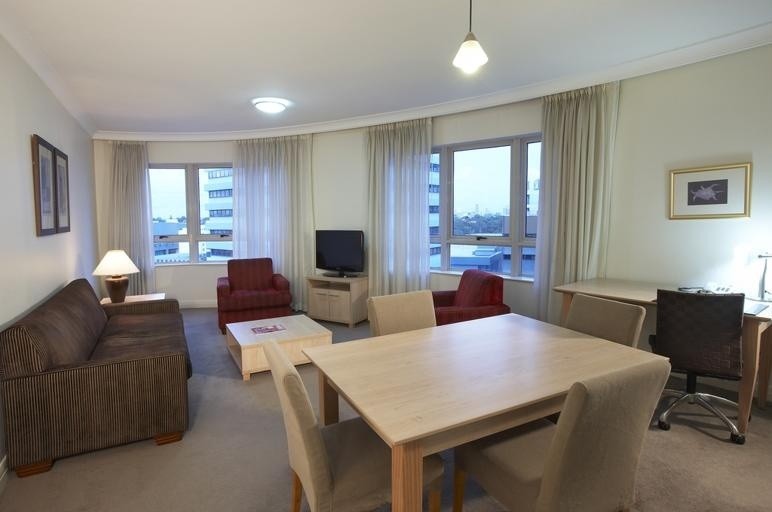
[250,97,292,116]
[450,0,488,76]
[90,248,143,304]
[746,250,771,304]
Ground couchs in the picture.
[1,278,194,482]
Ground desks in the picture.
[299,312,670,511]
[551,276,770,435]
[99,292,165,306]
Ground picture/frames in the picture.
[32,133,59,238]
[53,144,71,234]
[668,162,749,218]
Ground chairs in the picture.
[451,359,670,511]
[364,287,439,338]
[544,293,648,428]
[647,287,753,444]
[432,269,512,329]
[262,337,445,511]
[215,257,295,335]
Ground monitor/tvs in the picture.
[314,229,365,278]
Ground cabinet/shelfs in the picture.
[304,273,371,329]
